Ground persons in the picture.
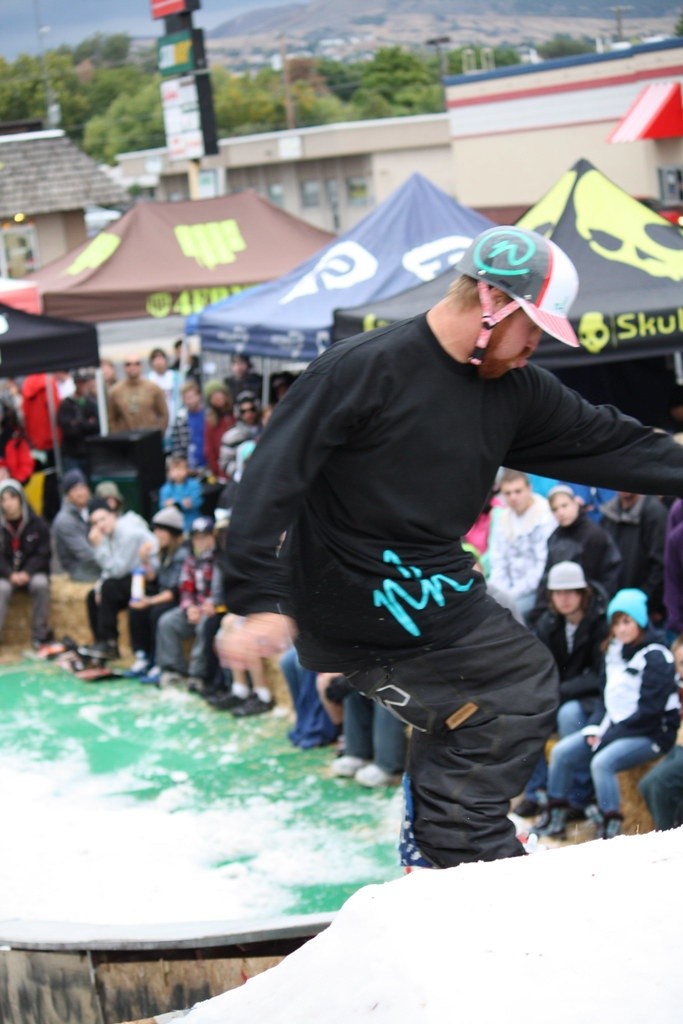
[0,338,306,718]
[461,427,682,841]
[279,646,404,787]
[219,227,683,872]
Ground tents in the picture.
[0,298,109,477]
[328,155,683,373]
[22,188,344,437]
[183,168,499,406]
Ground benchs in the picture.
[0,573,667,841]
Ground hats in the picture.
[151,505,184,528]
[455,225,580,348]
[607,587,648,629]
[61,469,86,492]
[547,559,588,590]
[94,481,124,504]
[74,367,95,381]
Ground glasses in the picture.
[239,407,256,414]
[124,361,142,367]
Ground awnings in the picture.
[609,80,683,142]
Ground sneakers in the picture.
[77,634,119,661]
[65,656,112,679]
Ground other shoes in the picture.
[207,691,249,709]
[356,764,394,787]
[235,692,276,718]
[137,663,168,686]
[331,754,371,777]
[529,802,570,841]
[125,656,150,677]
[594,813,623,841]
